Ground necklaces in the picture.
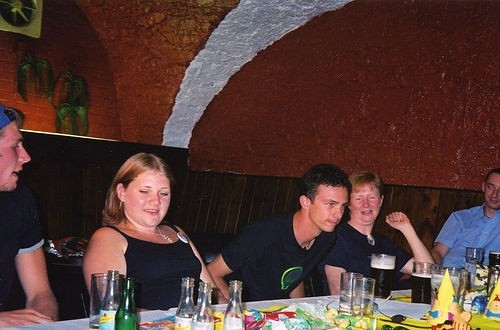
[134,225,173,244]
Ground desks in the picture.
[0,295,428,330]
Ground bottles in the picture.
[98,269,123,330]
[174,277,198,329]
[114,277,138,330]
[221,280,246,330]
[191,282,215,330]
[457,268,470,310]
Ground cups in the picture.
[339,271,364,317]
[351,277,375,320]
[370,253,397,300]
[410,261,441,305]
[88,273,126,330]
[440,266,468,299]
[464,246,485,269]
[486,251,500,297]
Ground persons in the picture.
[207,162,352,304]
[335,169,440,298]
[81,153,230,313]
[0,104,60,328]
[431,167,500,269]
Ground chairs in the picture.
[190,230,242,284]
[46,253,88,317]
